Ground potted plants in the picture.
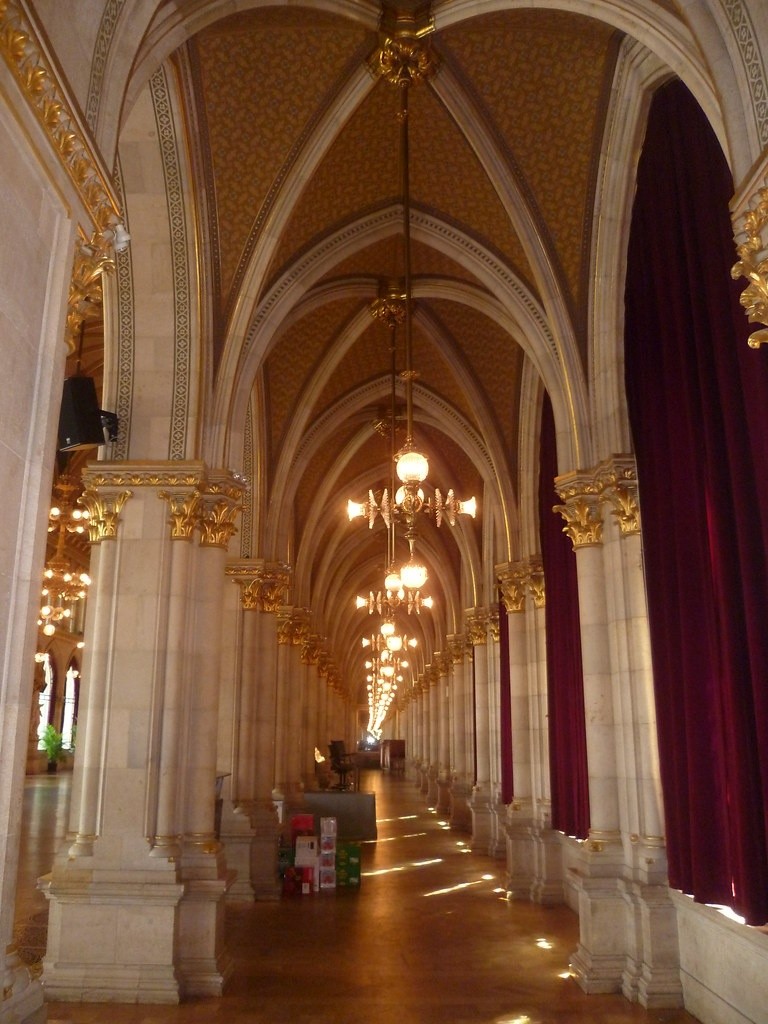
[44,725,63,774]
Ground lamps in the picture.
[36,473,91,635]
[57,376,119,455]
[346,38,479,738]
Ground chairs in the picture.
[329,740,358,789]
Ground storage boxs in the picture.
[277,815,361,895]
